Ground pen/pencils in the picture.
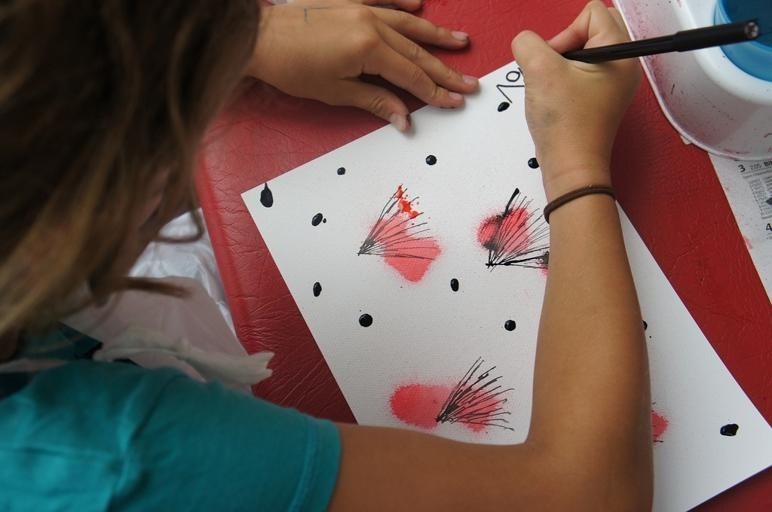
[563,17,760,63]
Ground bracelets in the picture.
[543,184,618,222]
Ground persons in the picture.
[0,0,658,512]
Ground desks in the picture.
[192,0,772,512]
